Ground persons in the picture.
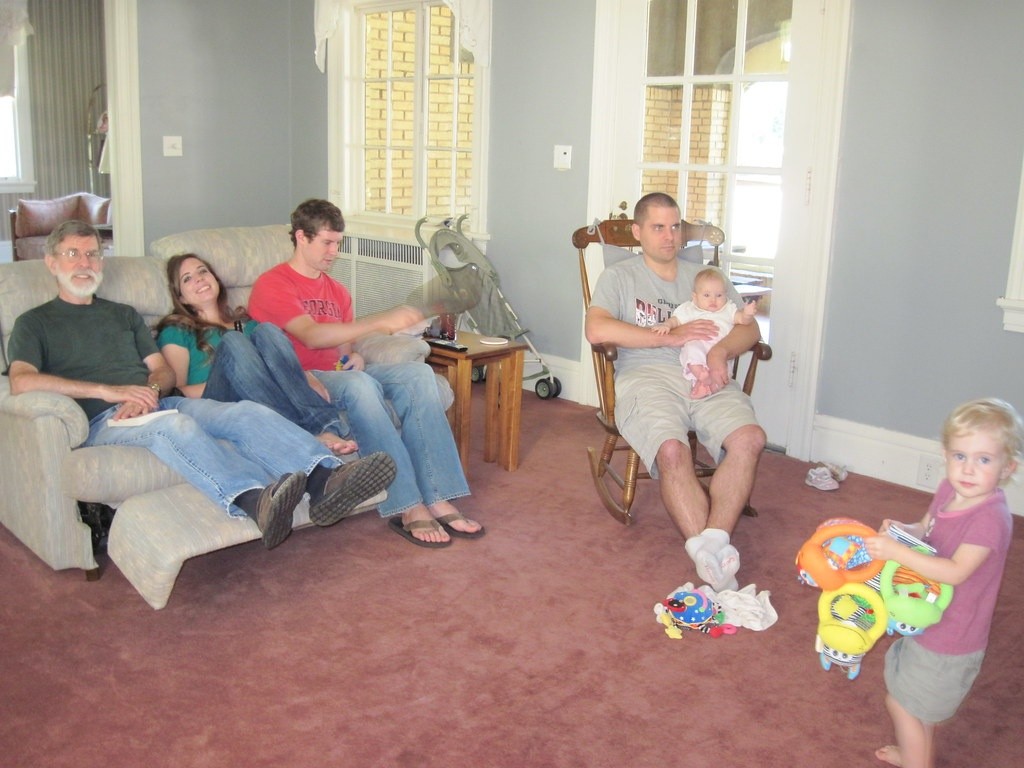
[585,193,760,590]
[873,395,1024,768]
[8,219,397,549]
[650,267,757,398]
[150,254,359,460]
[245,199,484,550]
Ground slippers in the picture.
[255,470,308,549]
[308,452,398,526]
[436,511,486,538]
[387,515,452,548]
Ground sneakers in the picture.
[815,461,848,482]
[805,467,840,490]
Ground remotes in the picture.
[425,339,468,353]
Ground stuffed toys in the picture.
[794,515,953,677]
[654,577,779,639]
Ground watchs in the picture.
[146,384,160,399]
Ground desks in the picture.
[734,284,771,302]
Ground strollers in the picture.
[415,214,562,400]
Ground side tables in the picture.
[425,329,528,482]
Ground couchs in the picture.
[10,193,113,259]
[0,257,389,608]
[151,224,455,436]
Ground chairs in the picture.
[573,220,772,526]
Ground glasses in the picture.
[51,248,104,263]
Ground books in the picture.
[108,406,178,428]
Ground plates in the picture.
[480,336,508,345]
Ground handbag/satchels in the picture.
[98,132,111,174]
